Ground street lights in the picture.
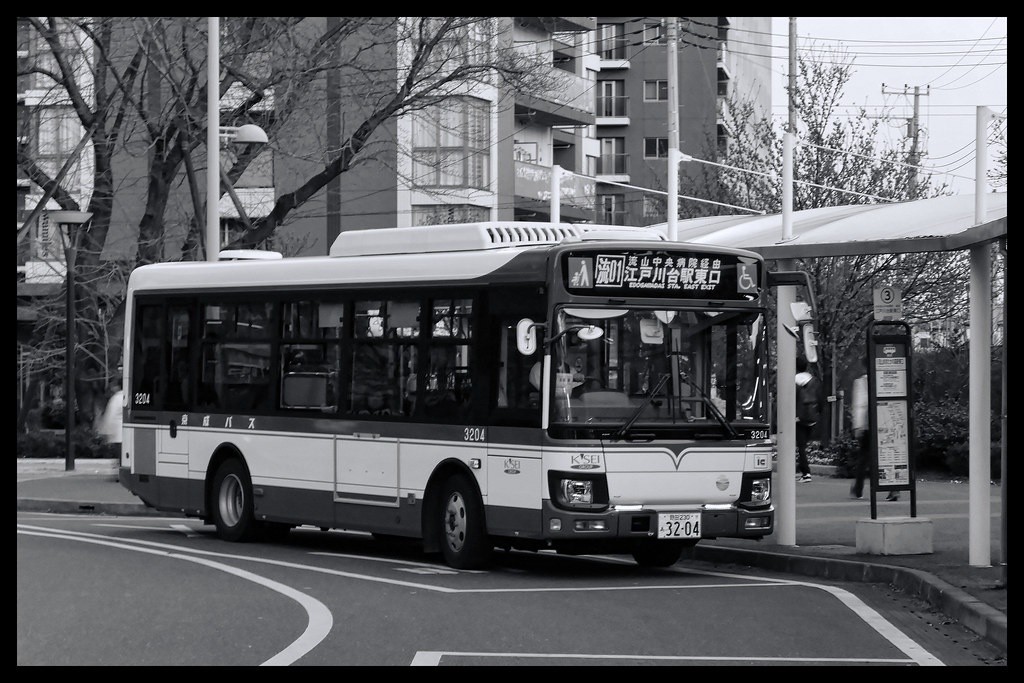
[205,122,269,264]
[47,205,94,476]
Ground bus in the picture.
[115,222,822,573]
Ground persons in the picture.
[846,355,901,502]
[358,385,392,416]
[796,354,827,484]
[710,371,722,419]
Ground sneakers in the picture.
[798,474,812,483]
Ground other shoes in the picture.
[850,489,863,499]
[885,492,900,500]
